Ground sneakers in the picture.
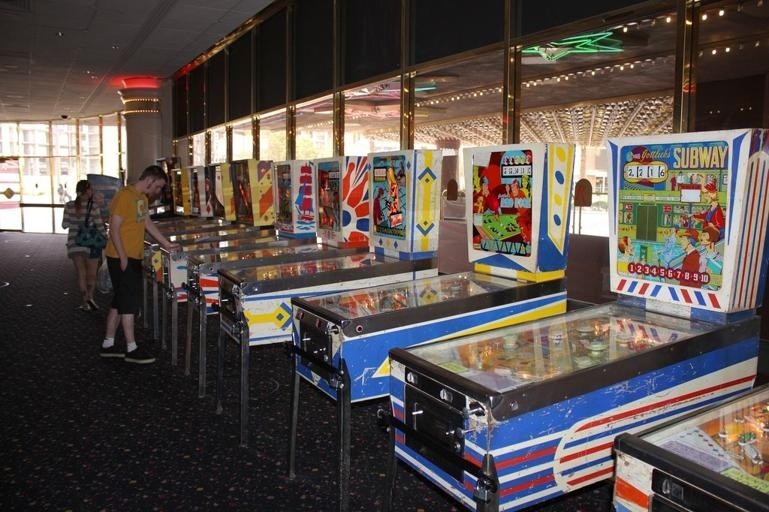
[98,344,157,365]
[73,297,100,312]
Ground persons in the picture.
[56,183,64,203]
[62,183,73,203]
[97,163,183,366]
[60,179,109,312]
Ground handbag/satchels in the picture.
[75,223,107,248]
